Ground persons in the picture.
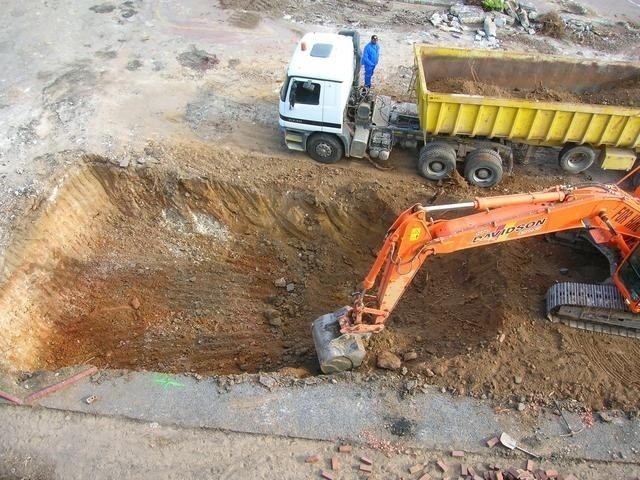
[361,34,380,90]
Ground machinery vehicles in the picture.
[309,166,640,375]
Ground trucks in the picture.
[278,29,640,188]
